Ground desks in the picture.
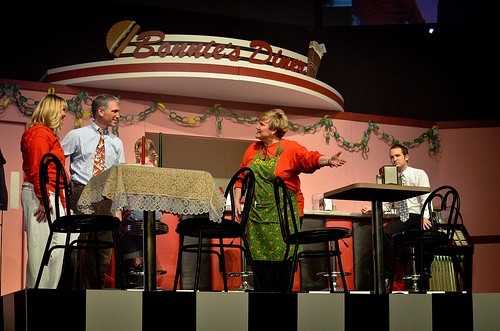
[324,184,431,294]
[76,164,227,291]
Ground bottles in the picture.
[332,204,336,211]
[398,177,403,185]
[376,174,383,184]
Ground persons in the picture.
[361,143,433,293]
[20,94,70,289]
[233,109,348,291]
[122,138,158,271]
[60,93,126,289]
[0,81,9,211]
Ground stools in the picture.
[124,220,169,291]
[230,228,254,291]
[401,247,423,293]
[316,229,353,291]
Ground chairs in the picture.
[273,176,351,294]
[34,153,125,291]
[173,167,255,291]
[392,185,460,295]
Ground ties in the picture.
[93,130,106,177]
[398,173,410,223]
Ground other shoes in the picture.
[133,262,144,272]
